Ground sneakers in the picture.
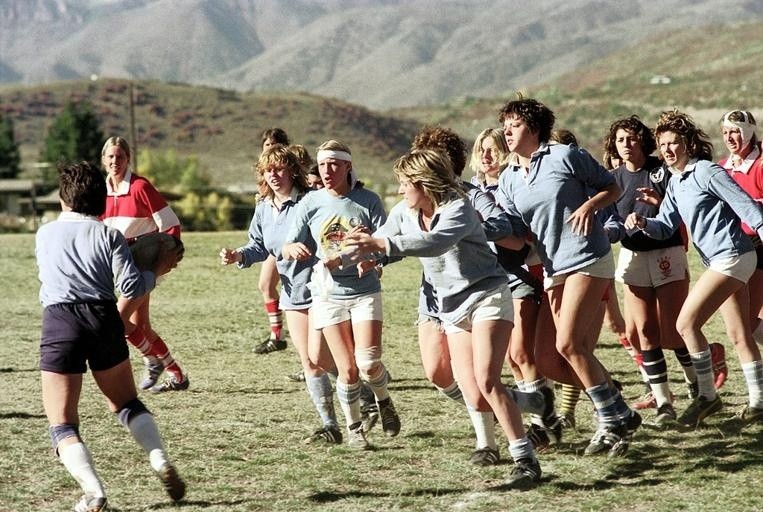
[251,339,287,353]
[347,432,367,450]
[676,394,722,432]
[284,369,305,382]
[526,423,549,449]
[656,404,676,422]
[585,423,627,454]
[685,375,698,398]
[140,360,163,389]
[361,395,378,432]
[508,458,541,488]
[712,343,728,389]
[607,412,641,458]
[149,376,188,392]
[724,405,763,424]
[536,386,561,442]
[303,427,341,444]
[69,494,107,512]
[377,396,400,437]
[469,446,500,467]
[557,414,575,429]
[633,390,674,409]
[156,462,185,501]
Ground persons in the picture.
[221,95,763,495]
[33,160,186,512]
[98,135,190,394]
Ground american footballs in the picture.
[129,232,177,271]
